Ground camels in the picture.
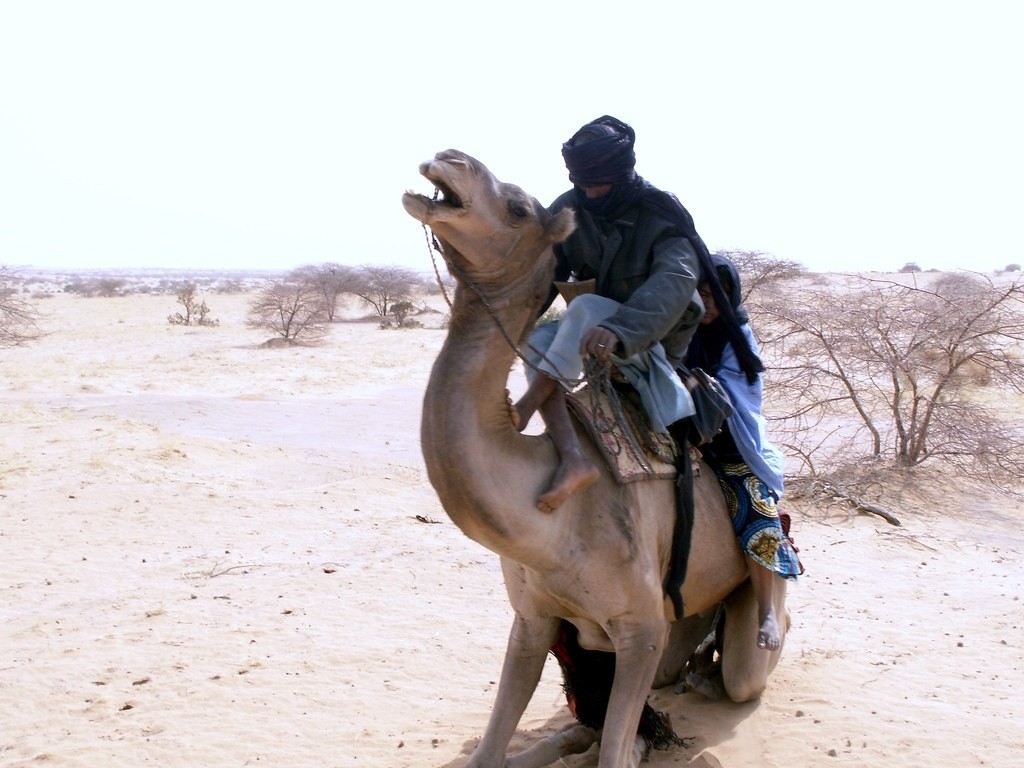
[401,146,793,768]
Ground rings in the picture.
[598,344,605,348]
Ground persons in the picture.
[696,255,800,652]
[504,116,764,514]
